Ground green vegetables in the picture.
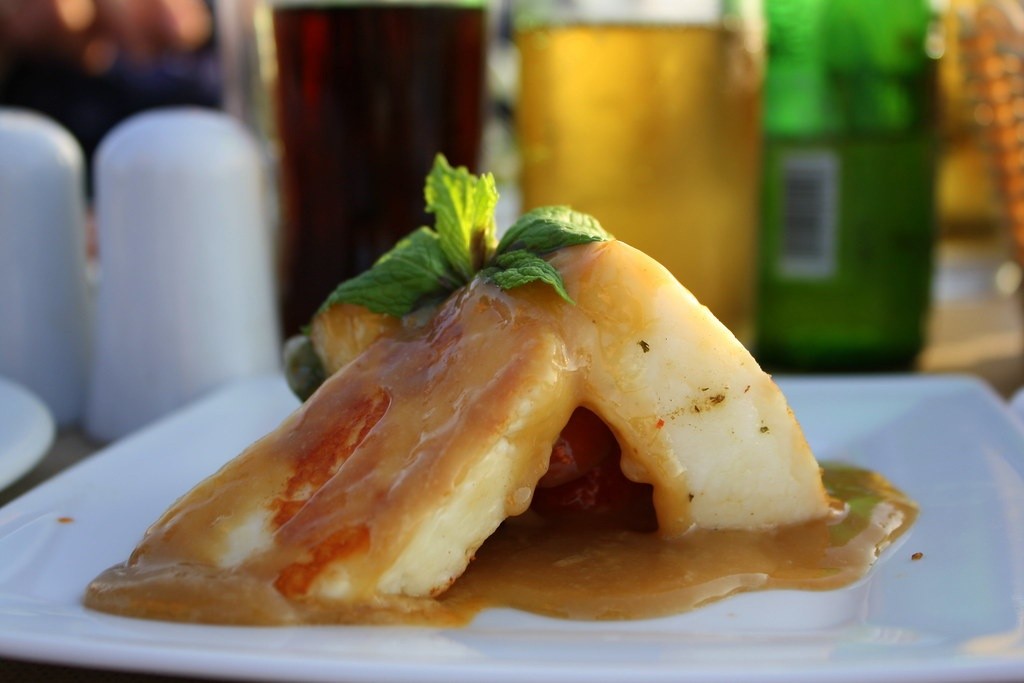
[298,150,613,334]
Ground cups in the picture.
[509,0,766,363]
[273,1,488,367]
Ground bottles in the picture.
[754,2,944,374]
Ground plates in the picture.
[0,375,57,493]
[0,374,1023,683]
